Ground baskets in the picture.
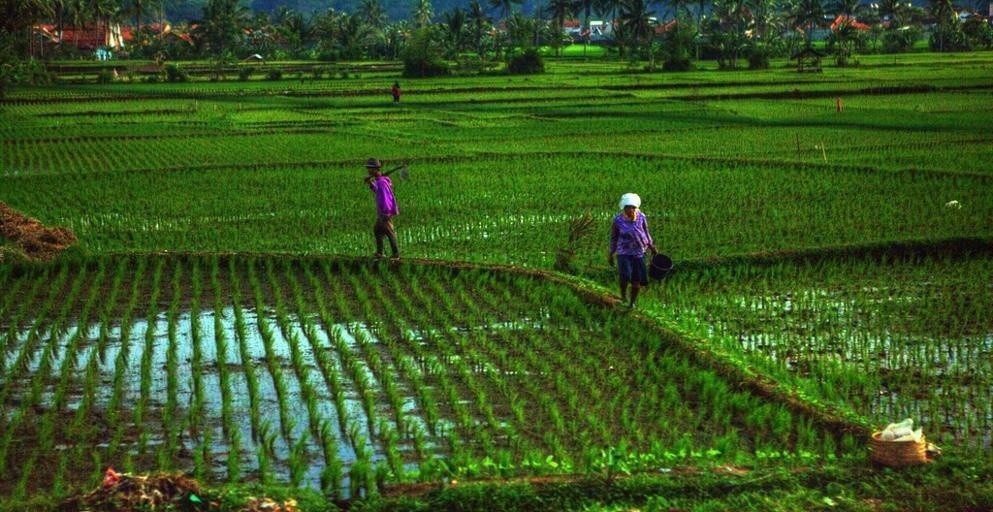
[872,430,927,466]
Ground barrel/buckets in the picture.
[649,255,673,280]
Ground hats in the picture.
[363,158,380,168]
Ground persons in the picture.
[390,80,401,103]
[363,158,400,261]
[608,193,656,308]
